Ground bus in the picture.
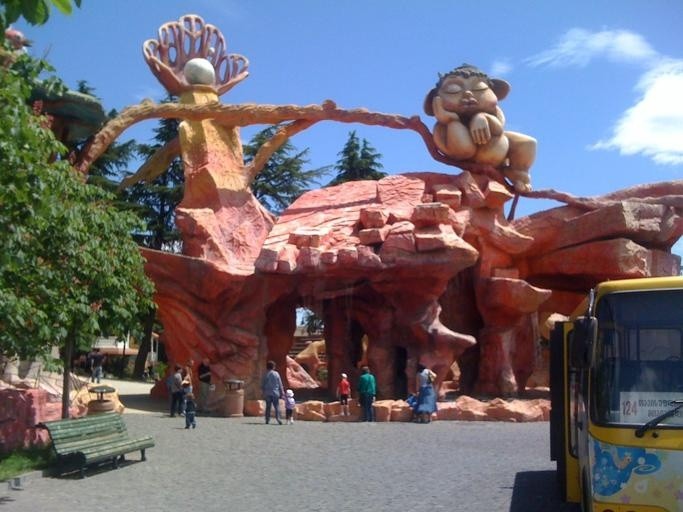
[548,274,683,512]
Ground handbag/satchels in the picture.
[428,373,435,383]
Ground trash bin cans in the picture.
[224,379,244,416]
[87,386,116,415]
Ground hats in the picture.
[286,390,295,397]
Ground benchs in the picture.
[37,410,155,481]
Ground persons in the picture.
[412,362,436,423]
[165,357,212,430]
[89,347,105,383]
[355,366,376,422]
[281,388,295,425]
[335,372,351,417]
[259,361,283,425]
[87,348,97,379]
